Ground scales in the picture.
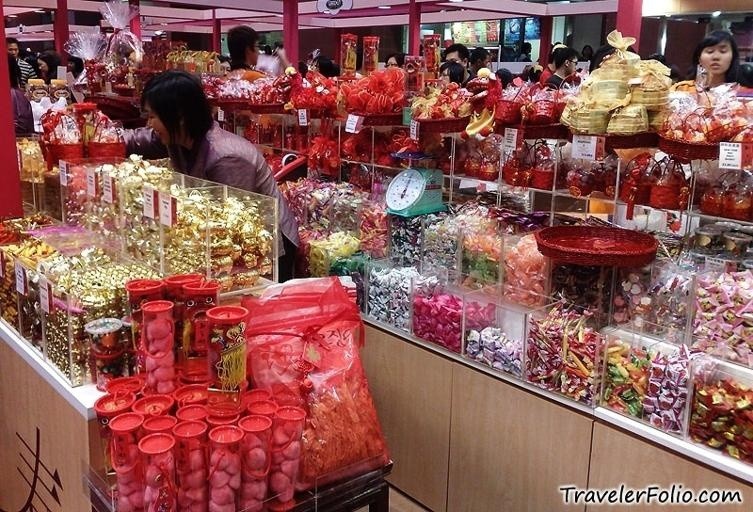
[385,152,446,218]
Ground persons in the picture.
[4,38,58,89]
[5,51,35,134]
[208,24,752,101]
[117,70,299,286]
[67,54,87,84]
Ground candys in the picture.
[0,33,752,459]
